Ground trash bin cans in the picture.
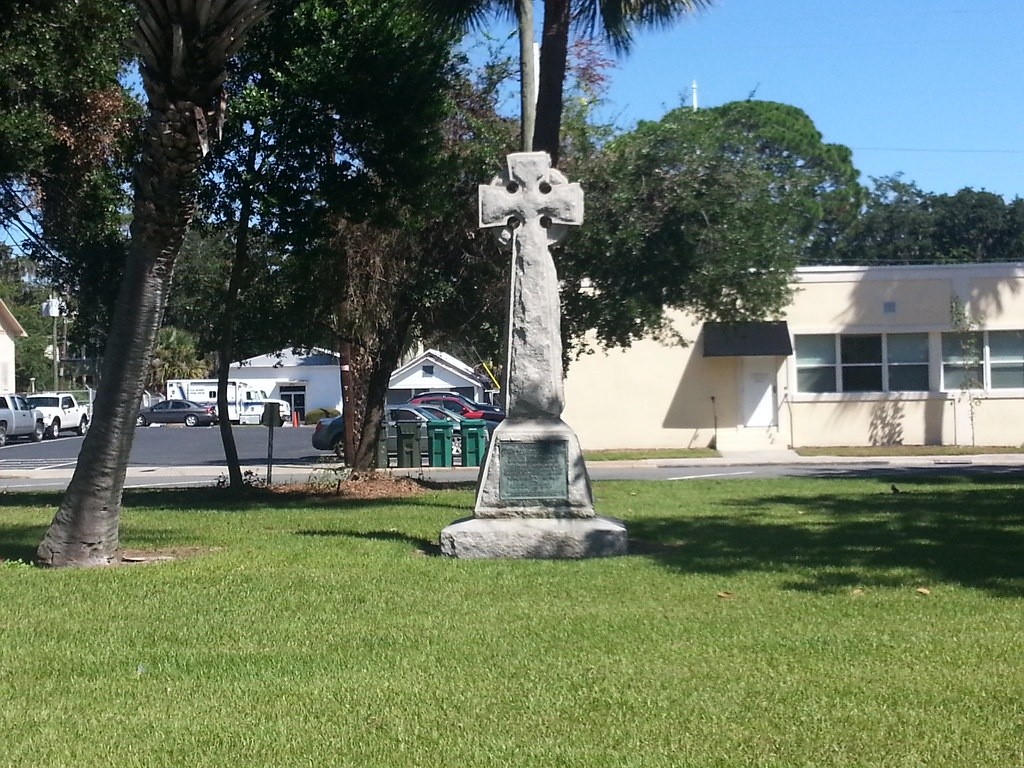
[395,419,423,468]
[460,419,487,467]
[426,420,454,467]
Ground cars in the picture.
[311,390,507,464]
[134,399,219,427]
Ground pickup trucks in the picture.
[22,391,90,439]
[0,395,45,449]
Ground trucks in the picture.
[166,378,289,428]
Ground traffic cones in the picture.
[291,411,298,427]
